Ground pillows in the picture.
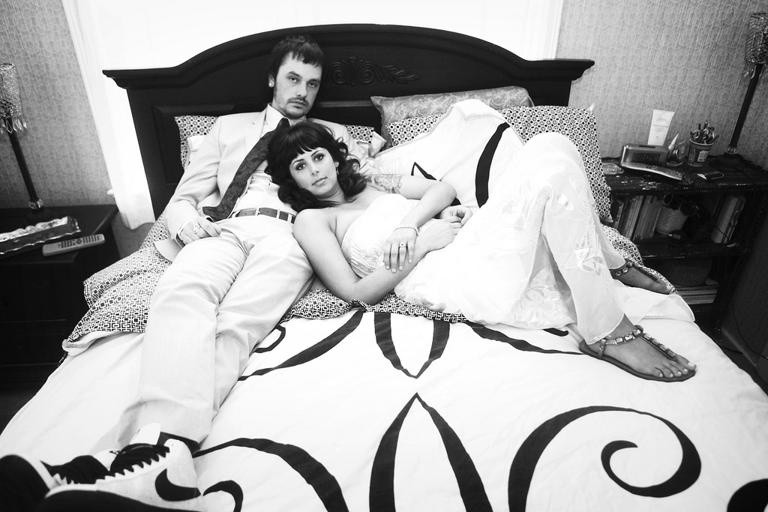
[385,105,615,224]
[370,86,534,137]
[174,116,374,172]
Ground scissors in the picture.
[691,130,708,143]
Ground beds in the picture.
[1,24,768,512]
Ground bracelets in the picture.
[391,224,417,234]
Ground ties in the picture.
[203,118,289,221]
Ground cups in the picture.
[687,138,714,168]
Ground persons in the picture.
[265,120,697,381]
[0,37,474,512]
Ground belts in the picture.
[228,208,295,223]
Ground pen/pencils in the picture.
[690,120,719,145]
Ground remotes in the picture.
[42,234,105,256]
[621,162,682,182]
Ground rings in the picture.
[399,243,406,248]
[193,224,201,235]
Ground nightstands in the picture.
[1,205,121,391]
[602,155,767,330]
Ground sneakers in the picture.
[0,424,200,512]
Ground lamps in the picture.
[717,12,768,168]
[0,63,51,225]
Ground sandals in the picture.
[610,257,670,295]
[580,322,697,381]
